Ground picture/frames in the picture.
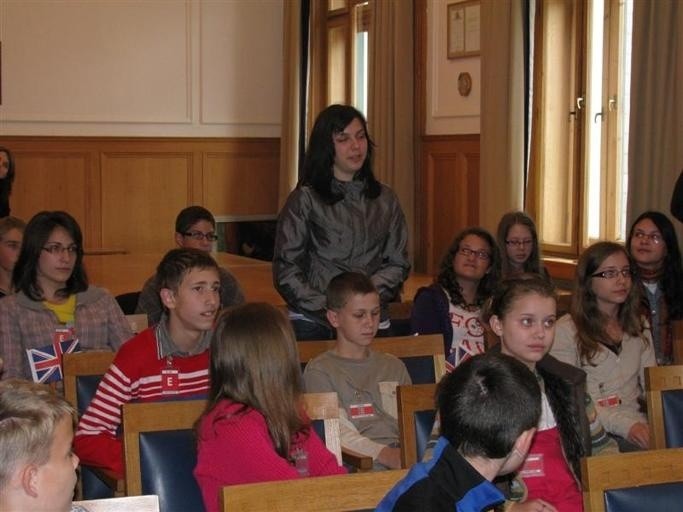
[446,0,481,61]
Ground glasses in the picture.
[38,245,78,255]
[632,232,664,244]
[458,247,491,260]
[503,239,535,248]
[588,269,637,280]
[176,229,218,241]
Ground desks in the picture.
[79,250,266,270]
[78,261,451,310]
[82,240,131,256]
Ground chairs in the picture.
[220,470,411,512]
[62,350,127,501]
[645,364,683,449]
[580,447,683,512]
[73,494,160,512]
[119,393,343,512]
[124,314,149,337]
[296,333,445,472]
[388,300,415,336]
[396,384,440,470]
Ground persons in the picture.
[557,242,661,452]
[192,301,349,512]
[0,145,16,219]
[626,210,682,430]
[274,104,414,339]
[414,228,497,375]
[670,170,682,225]
[480,271,620,512]
[0,216,29,297]
[497,210,545,275]
[1,210,134,389]
[134,206,245,322]
[74,247,223,479]
[304,271,427,472]
[0,377,79,512]
[376,354,542,512]
